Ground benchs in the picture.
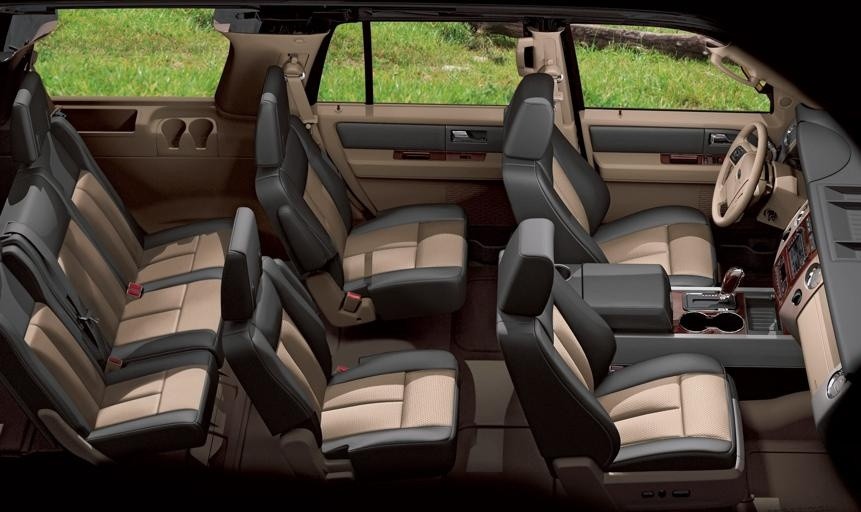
[1,71,232,459]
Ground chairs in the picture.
[250,66,469,322]
[502,72,717,288]
[219,204,459,482]
[497,216,747,502]
